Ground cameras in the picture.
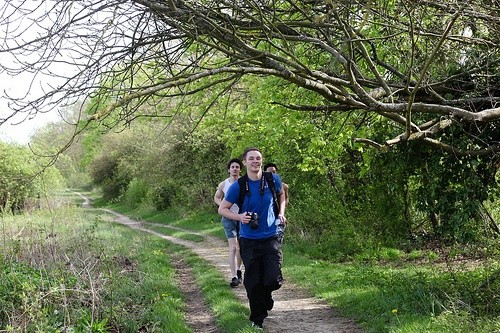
[246,213,260,230]
